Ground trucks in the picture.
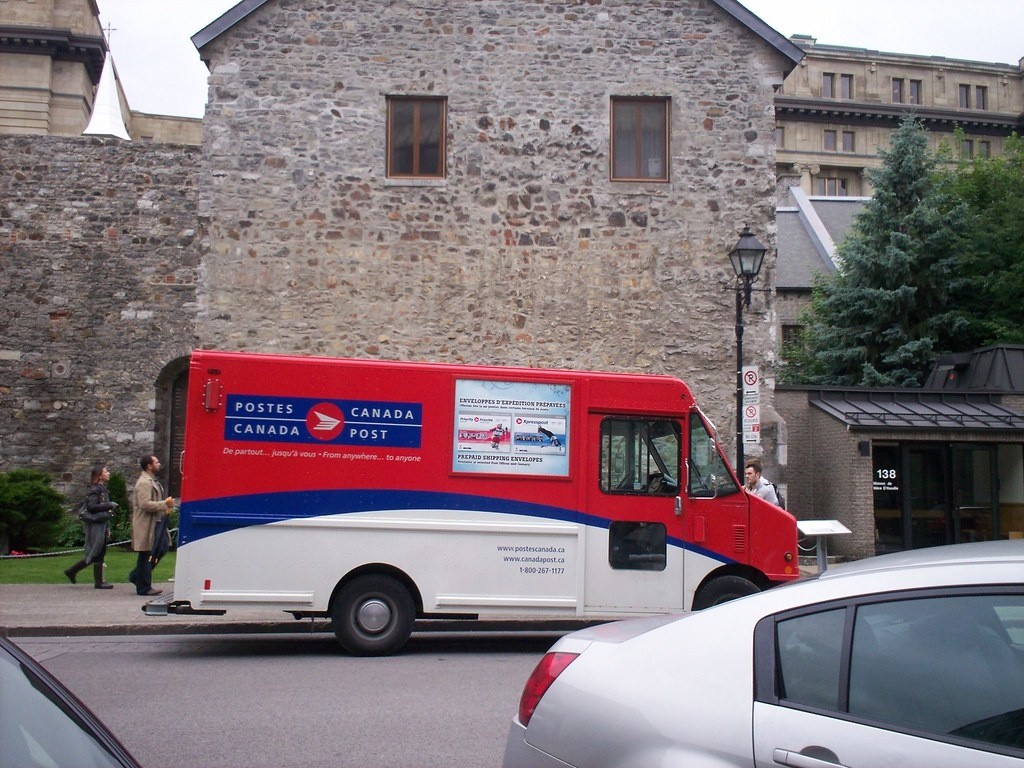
[143,349,797,657]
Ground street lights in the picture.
[723,224,772,486]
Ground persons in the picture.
[742,458,785,511]
[129,454,172,596]
[64,463,118,590]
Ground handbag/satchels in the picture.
[78,485,115,523]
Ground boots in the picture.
[64,560,86,584]
[94,562,114,589]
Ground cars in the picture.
[501,539,1023,768]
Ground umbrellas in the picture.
[148,499,174,563]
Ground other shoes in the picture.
[138,587,162,595]
